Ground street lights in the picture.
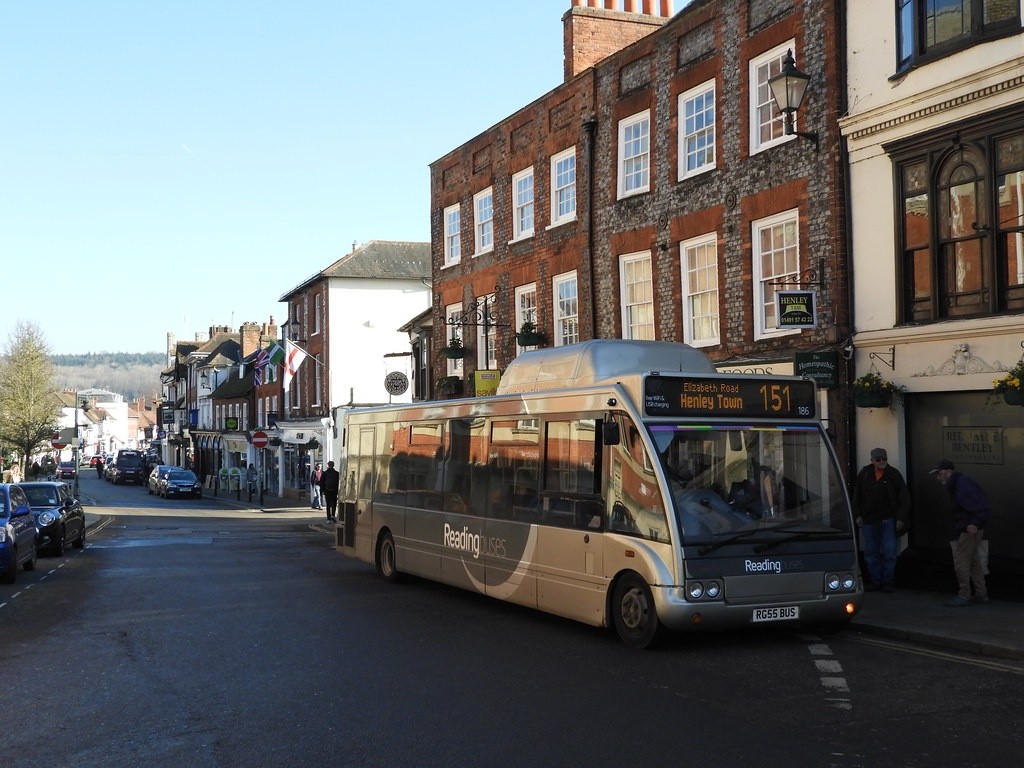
[74,392,90,496]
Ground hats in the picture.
[871,448,887,458]
[928,459,955,475]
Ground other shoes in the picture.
[947,598,970,607]
[327,519,337,524]
[319,506,323,510]
[972,595,990,603]
[868,580,882,589]
[884,580,897,593]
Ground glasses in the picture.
[874,458,887,462]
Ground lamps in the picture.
[200,372,211,391]
[768,49,819,151]
[290,317,306,347]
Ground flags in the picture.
[253,346,269,390]
[283,341,306,393]
[269,340,284,385]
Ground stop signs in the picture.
[51,433,67,449]
[252,432,268,448]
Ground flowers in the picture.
[983,360,1024,415]
[847,372,908,416]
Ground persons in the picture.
[143,458,165,488]
[6,464,23,483]
[747,465,782,520]
[32,461,40,481]
[852,448,912,593]
[928,460,993,606]
[320,461,339,524]
[310,463,325,510]
[246,463,258,496]
[96,457,104,479]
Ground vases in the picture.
[1003,388,1024,405]
[854,384,889,407]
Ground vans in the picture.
[111,449,146,486]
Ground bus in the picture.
[336,338,868,650]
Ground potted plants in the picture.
[436,337,466,361]
[268,437,281,446]
[437,376,463,394]
[305,437,318,449]
[506,322,548,347]
[168,440,184,448]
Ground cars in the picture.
[17,481,85,557]
[56,462,76,479]
[160,470,202,499]
[80,453,113,481]
[148,465,184,496]
[0,483,38,585]
